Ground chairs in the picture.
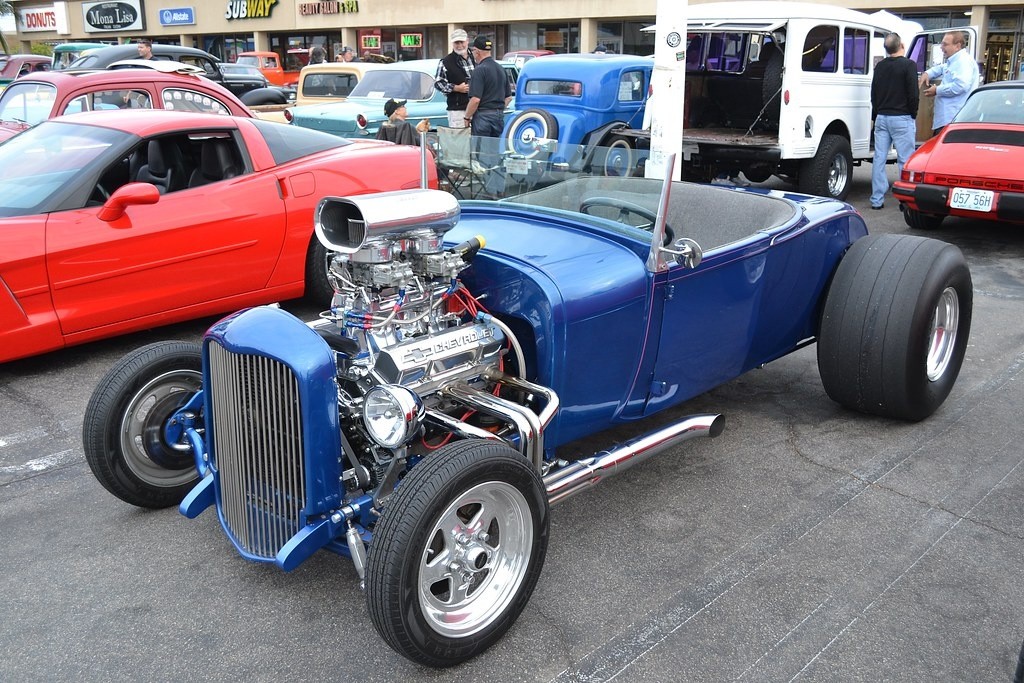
[136,137,238,195]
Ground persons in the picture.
[131,40,161,69]
[305,46,362,92]
[385,31,514,202]
[870,30,980,209]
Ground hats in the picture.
[339,46,353,55]
[450,28,467,43]
[384,98,408,118]
[473,36,493,51]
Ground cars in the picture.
[0,42,556,106]
[284,60,448,145]
[81,132,975,669]
[501,52,655,181]
[0,71,260,178]
[892,80,1024,229]
[0,108,439,357]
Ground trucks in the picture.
[643,2,980,201]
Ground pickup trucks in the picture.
[248,62,386,124]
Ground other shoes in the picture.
[872,202,884,210]
